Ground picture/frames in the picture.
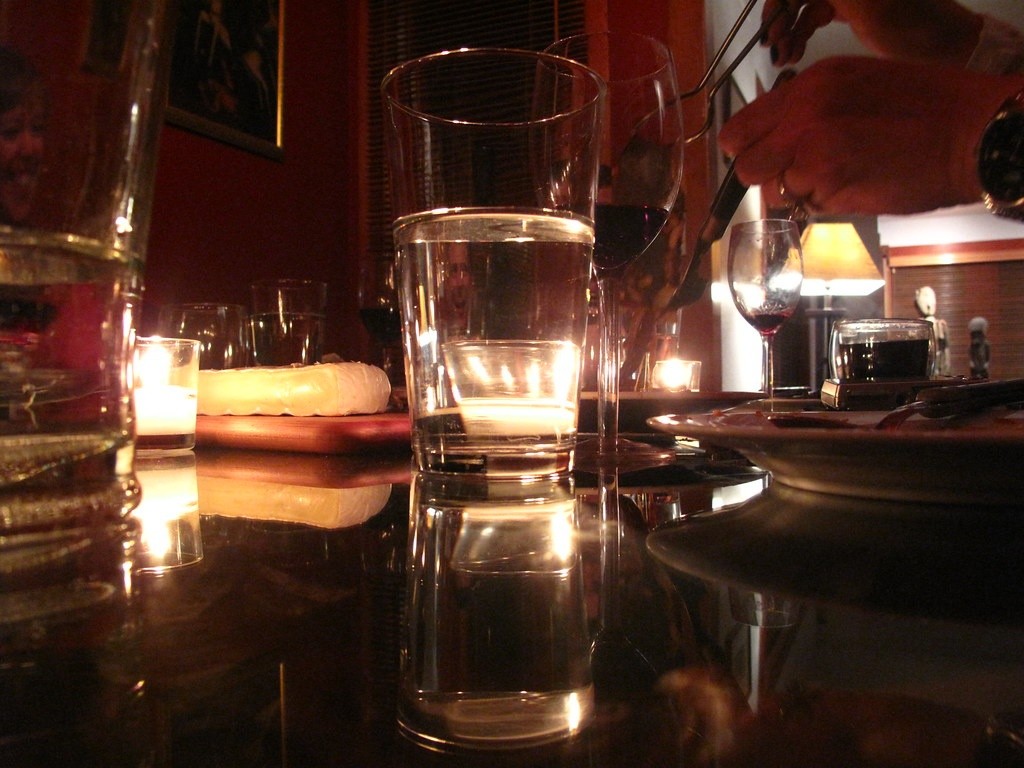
[74,0,285,164]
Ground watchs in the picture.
[970,92,1024,222]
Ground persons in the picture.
[966,317,992,378]
[718,1,1022,227]
[444,243,472,340]
[912,285,952,378]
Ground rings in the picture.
[791,203,813,224]
[775,172,801,206]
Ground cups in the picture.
[380,47,605,481]
[243,312,324,367]
[131,451,204,575]
[395,475,594,757]
[0,0,160,568]
[829,318,936,378]
[252,281,326,366]
[135,336,201,453]
[171,304,249,367]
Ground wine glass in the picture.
[535,31,683,473]
[727,220,804,418]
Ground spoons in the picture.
[665,69,799,311]
[768,379,1024,430]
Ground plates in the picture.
[647,399,1024,507]
[578,390,769,436]
